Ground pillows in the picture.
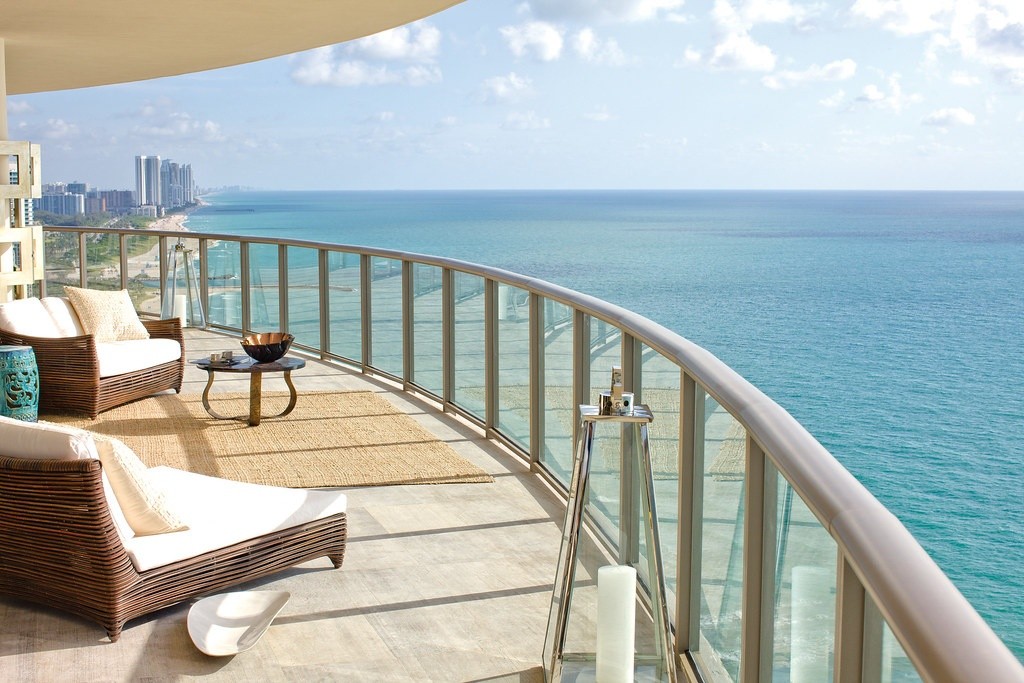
[0,296,63,338]
[64,285,152,342]
[0,416,135,544]
[38,420,191,536]
[39,297,86,337]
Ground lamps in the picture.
[169,295,187,327]
[595,563,636,683]
[789,564,832,683]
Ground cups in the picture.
[222,352,232,359]
[211,354,221,362]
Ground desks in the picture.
[186,354,306,426]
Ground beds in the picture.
[0,455,349,645]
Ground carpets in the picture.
[39,390,495,489]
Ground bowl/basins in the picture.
[240,332,294,363]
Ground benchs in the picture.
[0,316,185,419]
